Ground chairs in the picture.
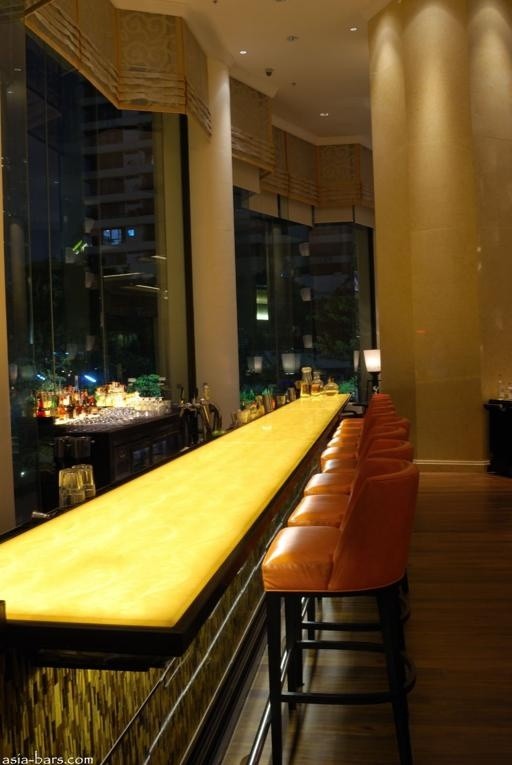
[261,392,420,765]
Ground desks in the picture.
[38,407,198,481]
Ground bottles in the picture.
[324,375,339,397]
[69,414,112,433]
[32,375,98,420]
[310,369,324,397]
[136,397,172,418]
[300,367,313,398]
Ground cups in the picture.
[100,408,141,429]
[58,464,96,509]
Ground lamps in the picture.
[362,349,385,392]
